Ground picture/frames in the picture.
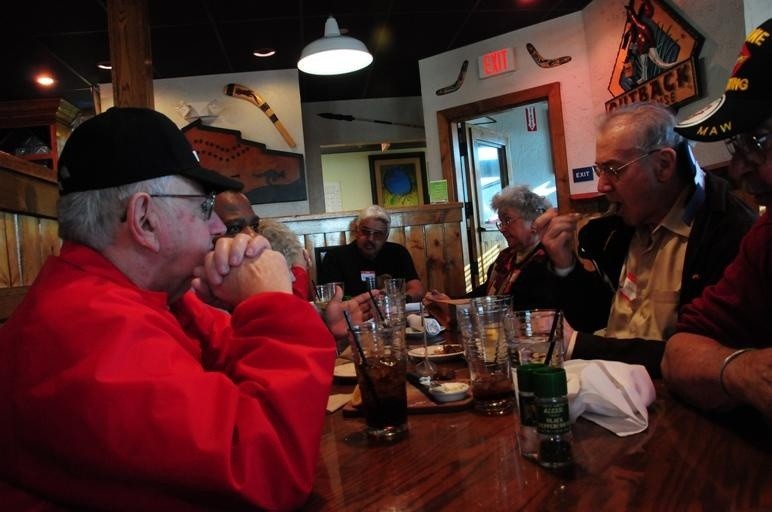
[367,151,430,209]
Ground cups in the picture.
[346,320,409,442]
[311,280,346,310]
[470,294,514,366]
[456,304,516,414]
[365,275,408,320]
[502,308,564,368]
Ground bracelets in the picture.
[719,347,761,402]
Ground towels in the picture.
[563,356,660,439]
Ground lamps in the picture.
[293,13,375,79]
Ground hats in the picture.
[57,107,245,195]
[674,19,770,141]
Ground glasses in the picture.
[495,216,520,228]
[723,131,772,160]
[359,228,386,239]
[594,148,661,183]
[119,193,217,223]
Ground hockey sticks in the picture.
[223,83,296,149]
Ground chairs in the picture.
[314,244,346,278]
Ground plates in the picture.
[405,326,446,338]
[333,362,357,377]
[431,382,470,401]
[408,344,465,361]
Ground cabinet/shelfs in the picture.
[0,98,82,176]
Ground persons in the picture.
[533,104,758,377]
[261,221,313,299]
[317,204,427,302]
[200,171,385,338]
[0,108,338,511]
[660,19,771,419]
[420,185,556,323]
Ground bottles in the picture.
[517,363,548,458]
[531,367,576,469]
